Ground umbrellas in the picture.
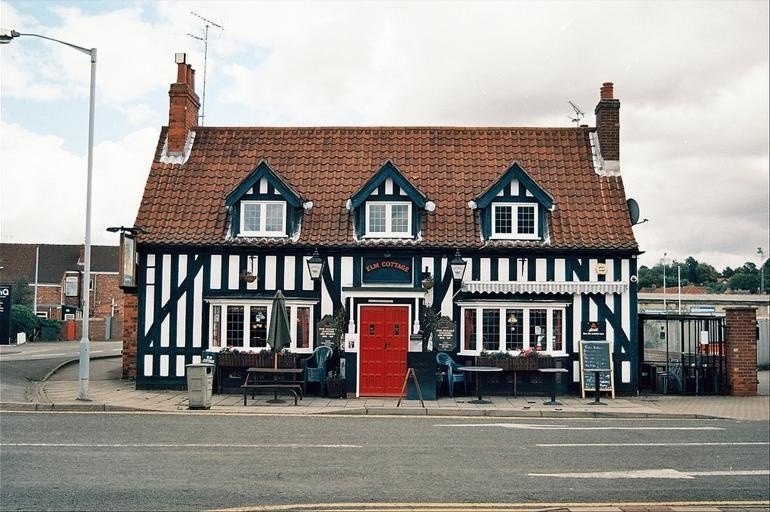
[266,289,291,403]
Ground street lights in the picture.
[756,244,766,294]
[0,30,100,402]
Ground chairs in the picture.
[657,357,684,394]
[436,351,467,396]
[303,345,334,396]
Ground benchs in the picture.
[248,380,306,399]
[241,383,301,406]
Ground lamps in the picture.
[449,249,467,281]
[306,247,327,280]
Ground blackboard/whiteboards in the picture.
[579,340,614,391]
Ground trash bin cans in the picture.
[185,364,216,410]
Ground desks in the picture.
[585,367,614,406]
[245,367,303,385]
[457,366,503,404]
[538,367,568,405]
